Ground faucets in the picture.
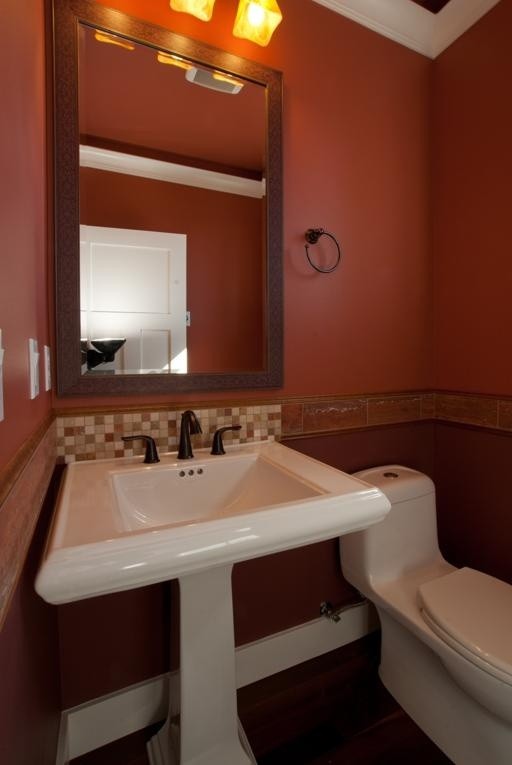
[177,409,203,459]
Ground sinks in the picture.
[34,438,390,604]
[91,337,127,362]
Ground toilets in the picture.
[338,463,512,765]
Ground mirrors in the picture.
[43,2,284,401]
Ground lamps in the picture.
[229,2,285,45]
[74,130,265,203]
[169,1,217,22]
